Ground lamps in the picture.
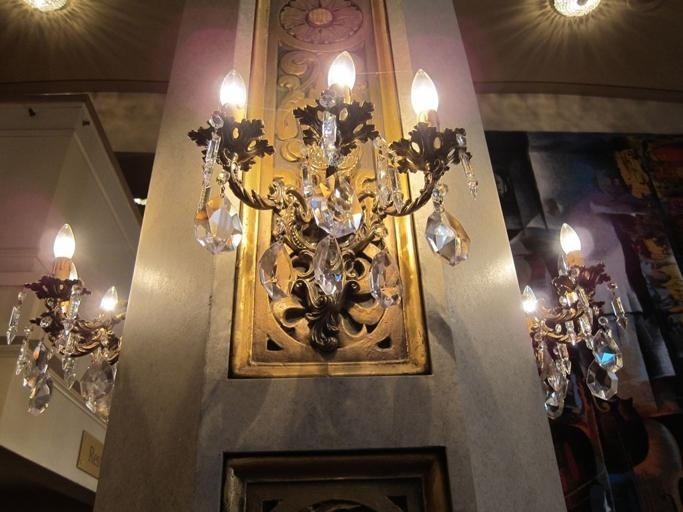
[7,223,127,422]
[187,49,479,354]
[521,222,631,422]
